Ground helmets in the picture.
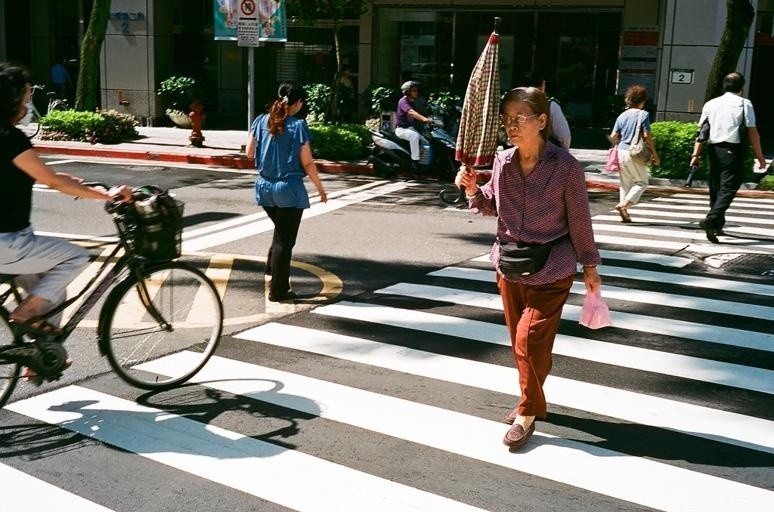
[401,81,418,94]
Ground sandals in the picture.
[8,316,62,335]
[22,360,72,388]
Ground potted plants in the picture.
[157,74,207,128]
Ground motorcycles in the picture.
[369,114,459,183]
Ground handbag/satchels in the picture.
[106,184,177,258]
[739,127,751,146]
[630,131,653,166]
[500,243,549,280]
[605,148,620,172]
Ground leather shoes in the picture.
[700,220,723,243]
[504,407,535,447]
[616,204,630,222]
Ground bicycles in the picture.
[14,83,69,139]
[0,181,224,409]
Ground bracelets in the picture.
[690,154,698,159]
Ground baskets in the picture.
[126,199,184,261]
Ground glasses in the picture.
[500,114,537,126]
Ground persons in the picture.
[240,78,328,303]
[521,71,572,149]
[610,83,662,224]
[0,58,135,378]
[338,64,358,99]
[687,70,768,246]
[452,86,602,452]
[393,79,435,173]
[49,57,73,100]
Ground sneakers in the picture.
[269,290,295,301]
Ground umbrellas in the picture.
[438,16,504,209]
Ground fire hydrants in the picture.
[187,100,204,146]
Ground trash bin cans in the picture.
[380,111,396,134]
